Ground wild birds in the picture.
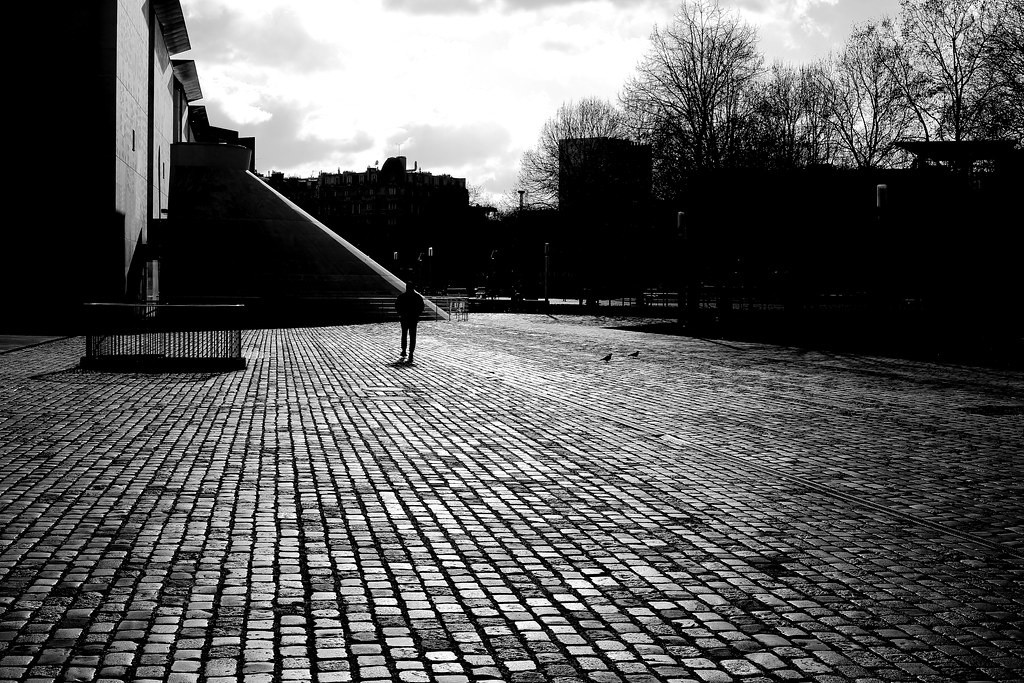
[628,351,641,359]
[599,353,614,364]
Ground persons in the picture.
[395,281,424,358]
